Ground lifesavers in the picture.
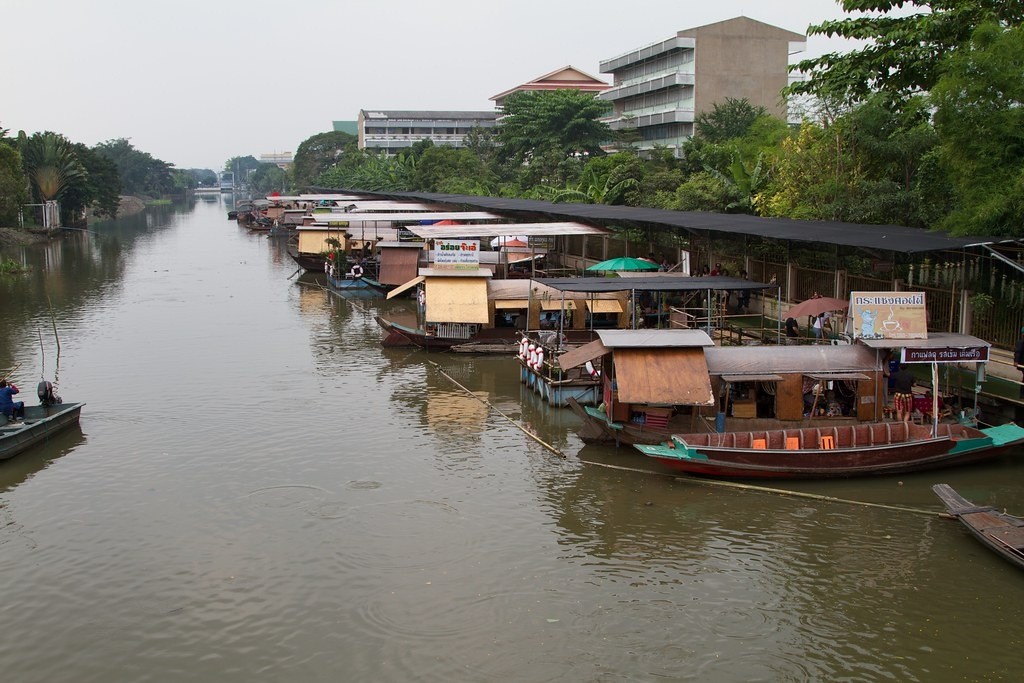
[534,347,544,371]
[546,333,569,342]
[274,219,278,228]
[330,266,333,276]
[325,261,328,273]
[418,290,425,307]
[519,338,528,360]
[527,344,536,368]
[351,265,363,278]
[586,361,601,376]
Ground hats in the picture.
[716,263,721,267]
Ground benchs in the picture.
[0,425,30,432]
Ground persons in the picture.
[0,378,24,421]
[361,242,370,257]
[274,201,328,209]
[812,311,832,342]
[495,309,569,328]
[692,262,747,319]
[785,317,801,345]
[885,354,917,422]
[639,291,653,309]
[826,390,842,416]
[343,233,356,250]
[430,239,434,250]
[376,237,383,255]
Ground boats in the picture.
[931,483,1024,574]
[0,380,86,462]
[557,328,993,447]
[237,193,782,409]
[633,419,1024,479]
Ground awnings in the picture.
[495,299,624,314]
[386,275,490,324]
[720,373,871,419]
[556,339,715,407]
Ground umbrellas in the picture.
[502,238,526,247]
[433,221,458,225]
[585,256,665,270]
[782,292,849,341]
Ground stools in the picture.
[910,414,924,425]
[941,405,953,419]
[883,406,893,418]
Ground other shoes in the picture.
[16,417,24,420]
[8,417,16,422]
